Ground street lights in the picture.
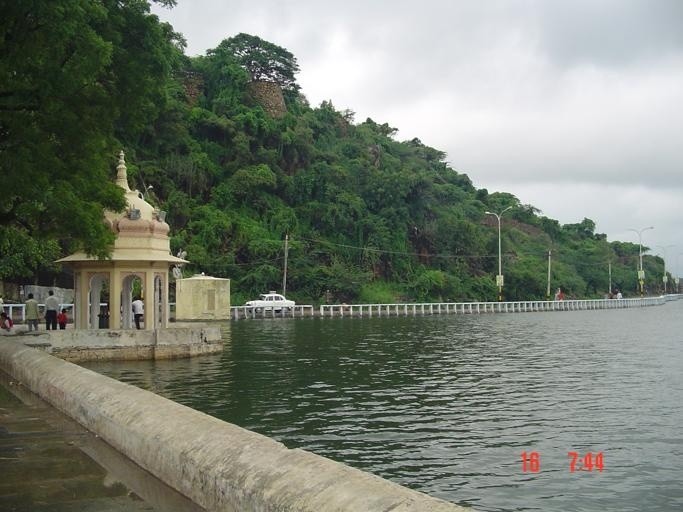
[656,244,674,292]
[628,225,654,297]
[485,204,513,301]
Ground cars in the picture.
[246,293,295,313]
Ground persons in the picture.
[616,290,622,300]
[131,296,144,330]
[557,291,565,300]
[0,313,13,332]
[57,308,67,330]
[43,290,59,330]
[24,293,40,336]
[0,294,4,314]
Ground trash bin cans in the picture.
[97,305,110,329]
